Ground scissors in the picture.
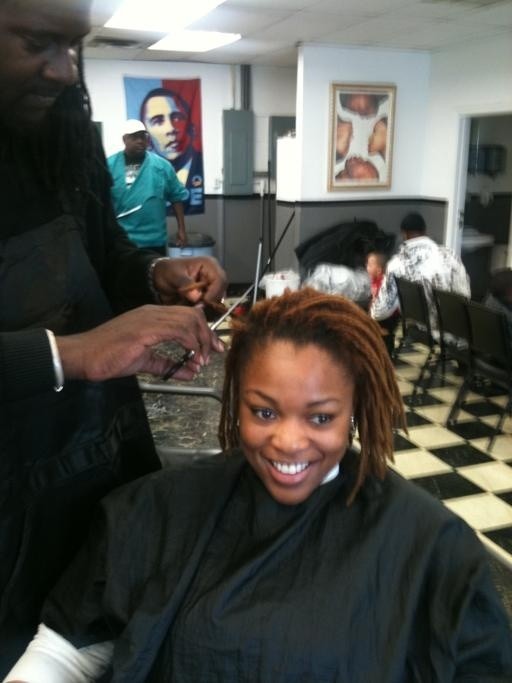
[156,274,255,385]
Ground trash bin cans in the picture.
[462,227,495,306]
[168,231,216,258]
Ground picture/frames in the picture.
[327,81,397,192]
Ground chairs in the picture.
[390,273,511,450]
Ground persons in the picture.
[333,88,388,183]
[0,289,512,683]
[365,211,471,354]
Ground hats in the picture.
[123,119,148,136]
